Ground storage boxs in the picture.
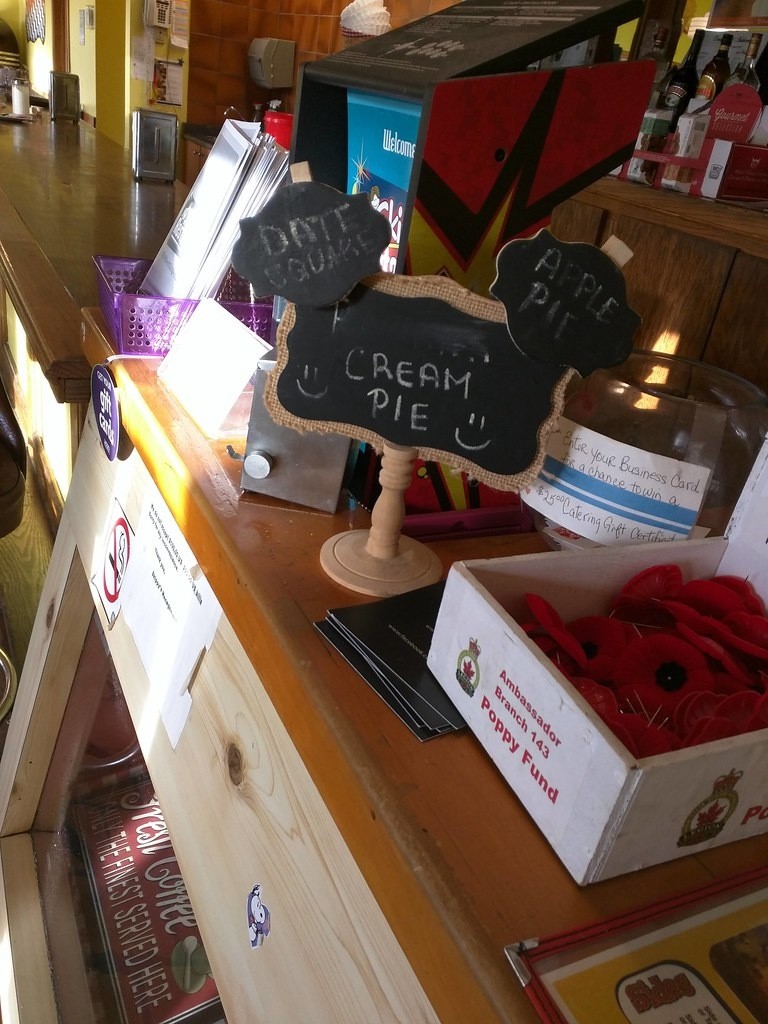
[427,429,768,888]
[610,97,768,214]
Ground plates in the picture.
[0,115,30,122]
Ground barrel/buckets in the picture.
[262,110,294,150]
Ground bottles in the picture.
[638,26,670,111]
[722,33,763,97]
[656,29,706,133]
[11,77,30,115]
[695,33,734,104]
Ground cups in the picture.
[519,349,767,558]
[29,105,43,115]
